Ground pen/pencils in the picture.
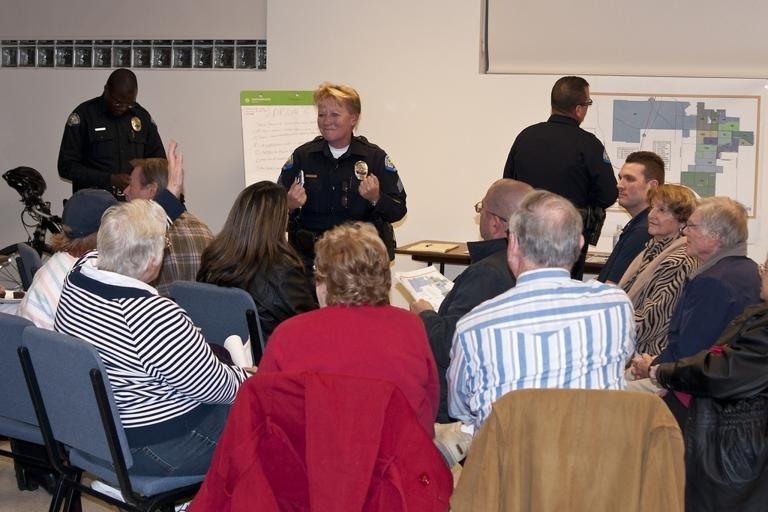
[299,170,304,215]
[426,243,432,247]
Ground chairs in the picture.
[15,242,42,291]
[0,313,70,512]
[185,370,454,512]
[17,325,207,512]
[168,279,265,367]
[449,388,687,512]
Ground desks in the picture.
[393,240,613,275]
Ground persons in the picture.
[626,196,762,394]
[501,74,619,280]
[257,222,443,440]
[443,190,638,440]
[604,182,697,361]
[16,190,120,333]
[631,254,768,511]
[9,437,72,495]
[595,147,666,284]
[53,138,246,476]
[123,157,216,296]
[56,69,169,201]
[412,176,538,423]
[196,180,318,369]
[277,83,410,276]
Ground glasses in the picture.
[475,202,508,223]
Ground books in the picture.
[407,242,459,254]
[394,263,455,314]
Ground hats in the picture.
[61,188,117,238]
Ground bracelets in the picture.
[371,199,378,207]
